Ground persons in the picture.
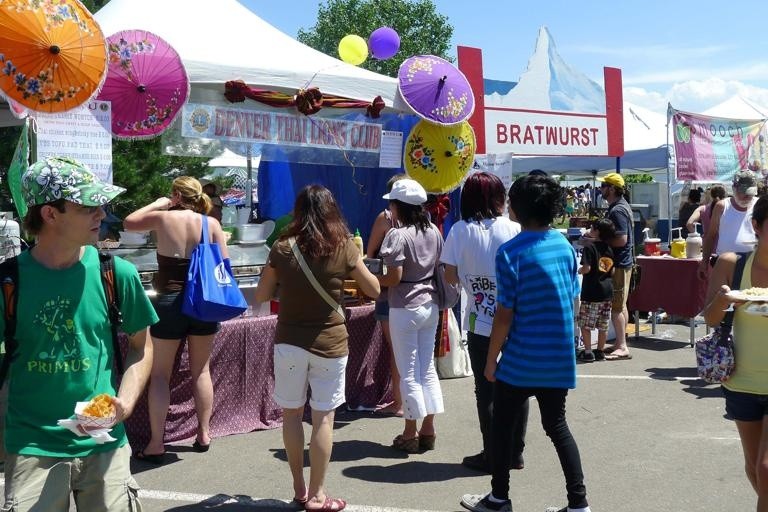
[124,175,230,460]
[677,167,767,511]
[0,155,161,511]
[255,184,380,511]
[435,168,586,512]
[365,173,446,452]
[574,173,636,363]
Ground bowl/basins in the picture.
[96,241,120,249]
[118,231,142,242]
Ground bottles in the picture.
[353,227,364,259]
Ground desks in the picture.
[115,303,393,443]
[632,256,710,348]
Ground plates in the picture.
[118,240,146,246]
[724,287,768,301]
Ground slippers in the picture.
[605,347,632,360]
[135,448,163,460]
[194,438,210,451]
[371,403,402,417]
[294,497,346,512]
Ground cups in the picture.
[368,258,380,273]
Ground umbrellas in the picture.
[397,54,475,125]
[0,1,109,115]
[86,28,189,143]
[403,118,477,197]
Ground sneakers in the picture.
[576,349,605,363]
[546,506,592,512]
[462,493,514,512]
[463,449,525,473]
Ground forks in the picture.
[721,301,737,312]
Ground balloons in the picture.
[337,34,368,67]
[369,26,401,59]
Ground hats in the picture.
[733,168,760,196]
[382,179,427,207]
[200,179,223,194]
[596,173,624,189]
[21,159,127,209]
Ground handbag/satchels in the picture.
[184,244,249,322]
[433,262,460,311]
[629,264,641,292]
[696,330,735,384]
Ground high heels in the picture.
[394,435,435,453]
